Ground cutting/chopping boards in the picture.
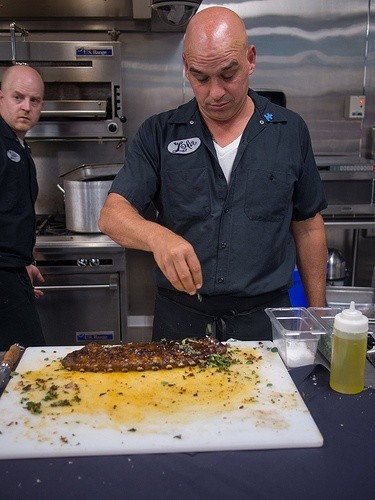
[1,340,324,460]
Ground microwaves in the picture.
[315,156,375,216]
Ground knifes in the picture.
[366,334,375,367]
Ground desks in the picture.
[0,347,375,500]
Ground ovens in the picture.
[0,40,124,141]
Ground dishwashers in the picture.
[31,274,127,344]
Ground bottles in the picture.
[330,301,368,394]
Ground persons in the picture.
[0,64,45,351]
[97,7,328,342]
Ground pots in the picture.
[57,179,115,233]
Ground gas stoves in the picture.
[34,213,124,273]
